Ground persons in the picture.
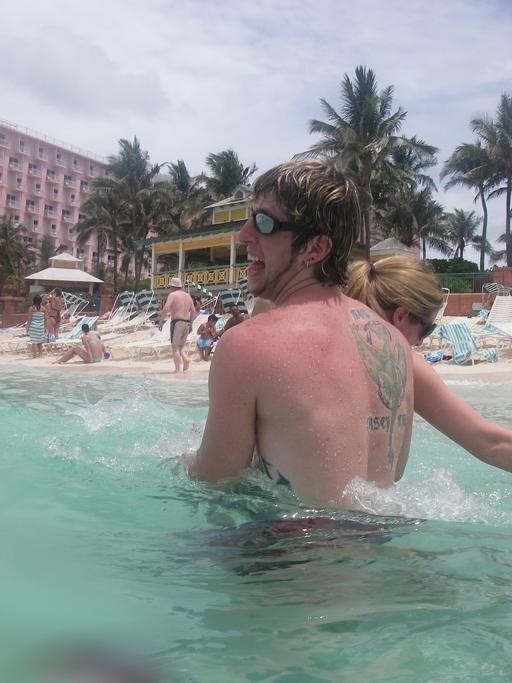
[171,156,413,514]
[197,314,219,359]
[25,287,107,363]
[191,295,202,313]
[217,303,245,336]
[344,254,511,472]
[158,277,195,371]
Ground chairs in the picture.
[3,277,254,362]
[426,281,512,366]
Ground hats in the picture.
[167,276,183,288]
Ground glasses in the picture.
[249,204,303,235]
[408,311,438,341]
